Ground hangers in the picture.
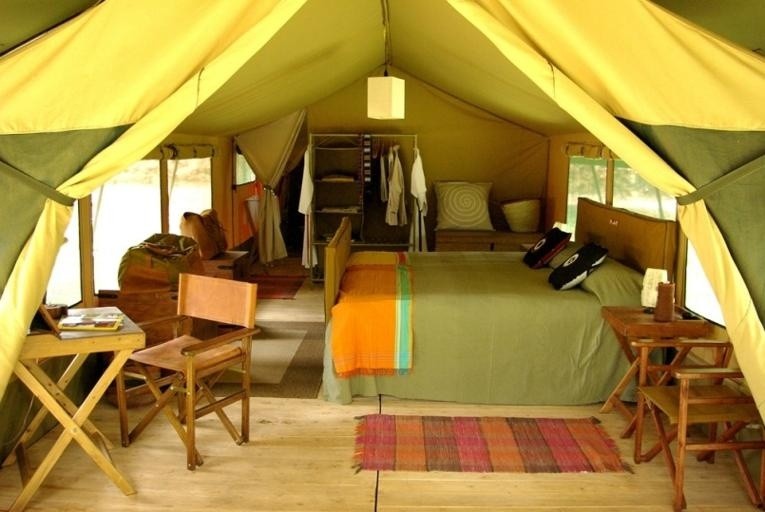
[373,136,401,161]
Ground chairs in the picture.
[630,337,765,512]
[113,273,261,471]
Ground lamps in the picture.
[367,19,406,121]
[641,268,668,314]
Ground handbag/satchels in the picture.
[179,208,228,260]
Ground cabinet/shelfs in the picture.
[200,251,248,280]
[97,283,218,407]
[306,133,418,284]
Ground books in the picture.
[58,312,126,333]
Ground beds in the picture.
[325,195,679,405]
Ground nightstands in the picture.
[521,243,534,250]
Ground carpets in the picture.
[351,412,636,473]
[242,273,310,298]
[205,318,326,398]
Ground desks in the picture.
[600,306,707,438]
[434,226,545,251]
[0,305,146,512]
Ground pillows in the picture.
[550,241,644,302]
[548,240,609,291]
[522,228,571,270]
[433,180,496,231]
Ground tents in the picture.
[0,0,764,512]
